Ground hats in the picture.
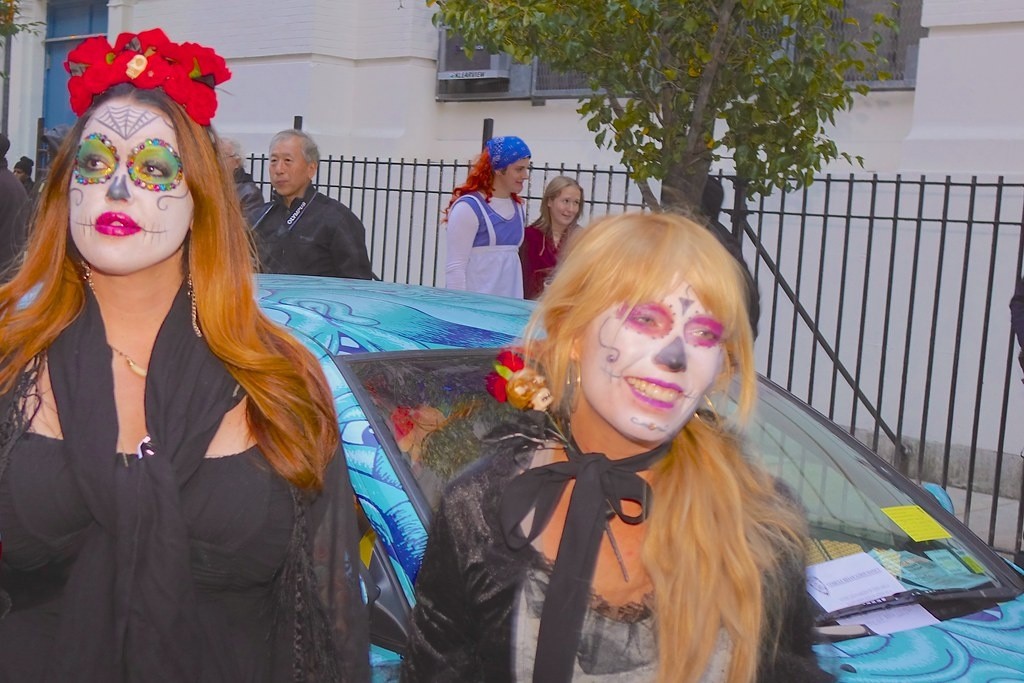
[14,156,33,175]
[0,133,10,157]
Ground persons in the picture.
[0,129,760,367]
[0,31,381,682]
[397,208,842,683]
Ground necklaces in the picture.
[78,263,147,380]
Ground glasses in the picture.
[224,152,234,157]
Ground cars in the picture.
[17,275,1024,683]
